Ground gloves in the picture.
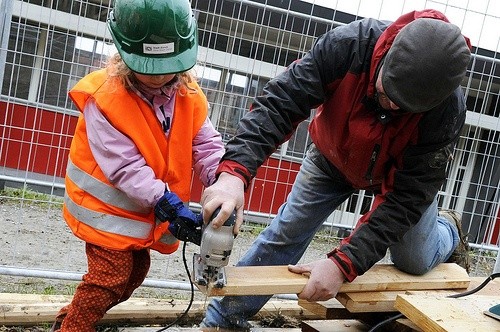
[155,191,205,245]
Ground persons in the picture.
[197,9,472,332]
[49,0,227,332]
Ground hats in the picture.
[385,18,472,113]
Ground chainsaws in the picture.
[192,197,237,288]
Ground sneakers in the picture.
[437,209,472,274]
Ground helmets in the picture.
[108,0,201,74]
